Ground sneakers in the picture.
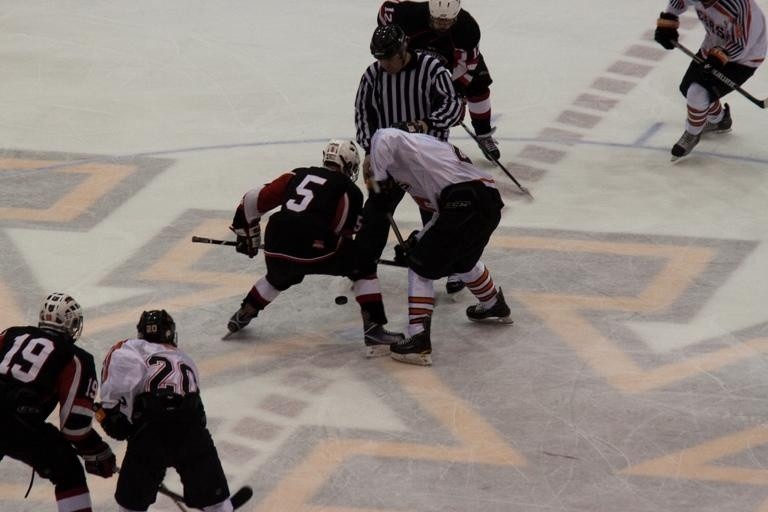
[700,103,732,134]
[466,286,508,320]
[672,130,700,157]
[228,302,259,332]
[445,278,465,295]
[391,336,433,356]
[363,324,405,345]
[476,126,500,161]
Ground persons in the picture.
[353,22,467,297]
[377,1,501,161]
[652,0,768,158]
[228,138,405,349]
[1,291,119,512]
[96,308,234,512]
[362,127,514,355]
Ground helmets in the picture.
[428,0,462,32]
[39,293,83,344]
[370,25,410,75]
[137,310,177,347]
[323,138,358,181]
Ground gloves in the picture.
[394,231,422,268]
[101,410,131,441]
[362,155,381,192]
[85,454,116,478]
[702,46,730,72]
[394,120,427,134]
[654,12,680,49]
[236,235,261,258]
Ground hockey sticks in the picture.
[673,38,768,109]
[109,464,253,512]
[459,121,532,198]
